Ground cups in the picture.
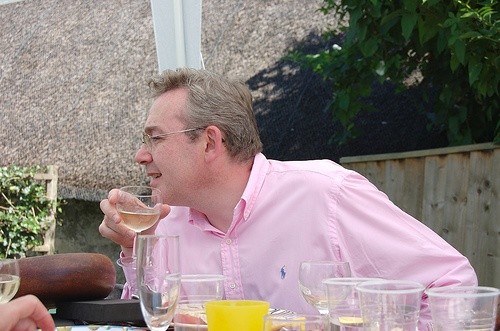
[205,300,269,331]
[424,286,500,331]
[263,315,322,331]
[323,277,387,331]
[132,235,180,331]
[174,274,223,331]
[355,281,424,331]
[0,257,21,306]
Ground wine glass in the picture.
[117,186,163,271]
[297,260,351,331]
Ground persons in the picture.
[99,69,478,331]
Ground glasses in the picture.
[141,127,226,157]
